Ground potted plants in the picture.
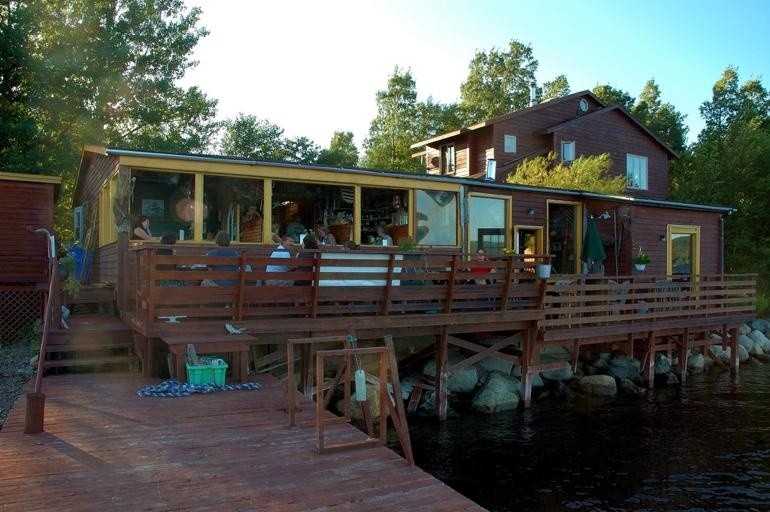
[632,254,651,272]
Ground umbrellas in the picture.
[579,216,606,274]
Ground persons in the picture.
[201,230,253,289]
[315,222,341,246]
[373,222,396,246]
[468,248,494,283]
[262,233,295,288]
[291,235,322,289]
[134,212,157,240]
[154,233,197,286]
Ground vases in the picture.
[536,262,552,278]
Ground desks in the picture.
[159,334,257,384]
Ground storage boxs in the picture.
[186,358,229,385]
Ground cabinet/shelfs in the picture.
[333,208,383,221]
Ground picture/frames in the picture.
[138,198,168,219]
